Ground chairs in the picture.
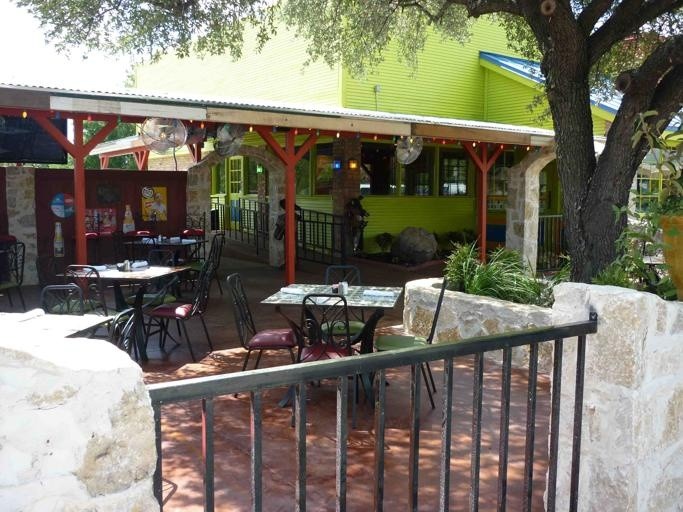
[363,279,447,409]
[291,294,357,430]
[225,273,297,399]
[0,241,27,311]
[320,265,366,352]
[41,230,225,363]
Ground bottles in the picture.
[124,260,130,271]
[123,205,135,234]
[339,281,348,295]
[86,208,114,232]
[53,222,64,258]
[158,235,161,241]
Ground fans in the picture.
[142,118,188,155]
[396,136,423,164]
[213,122,247,157]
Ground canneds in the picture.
[338,281,349,295]
